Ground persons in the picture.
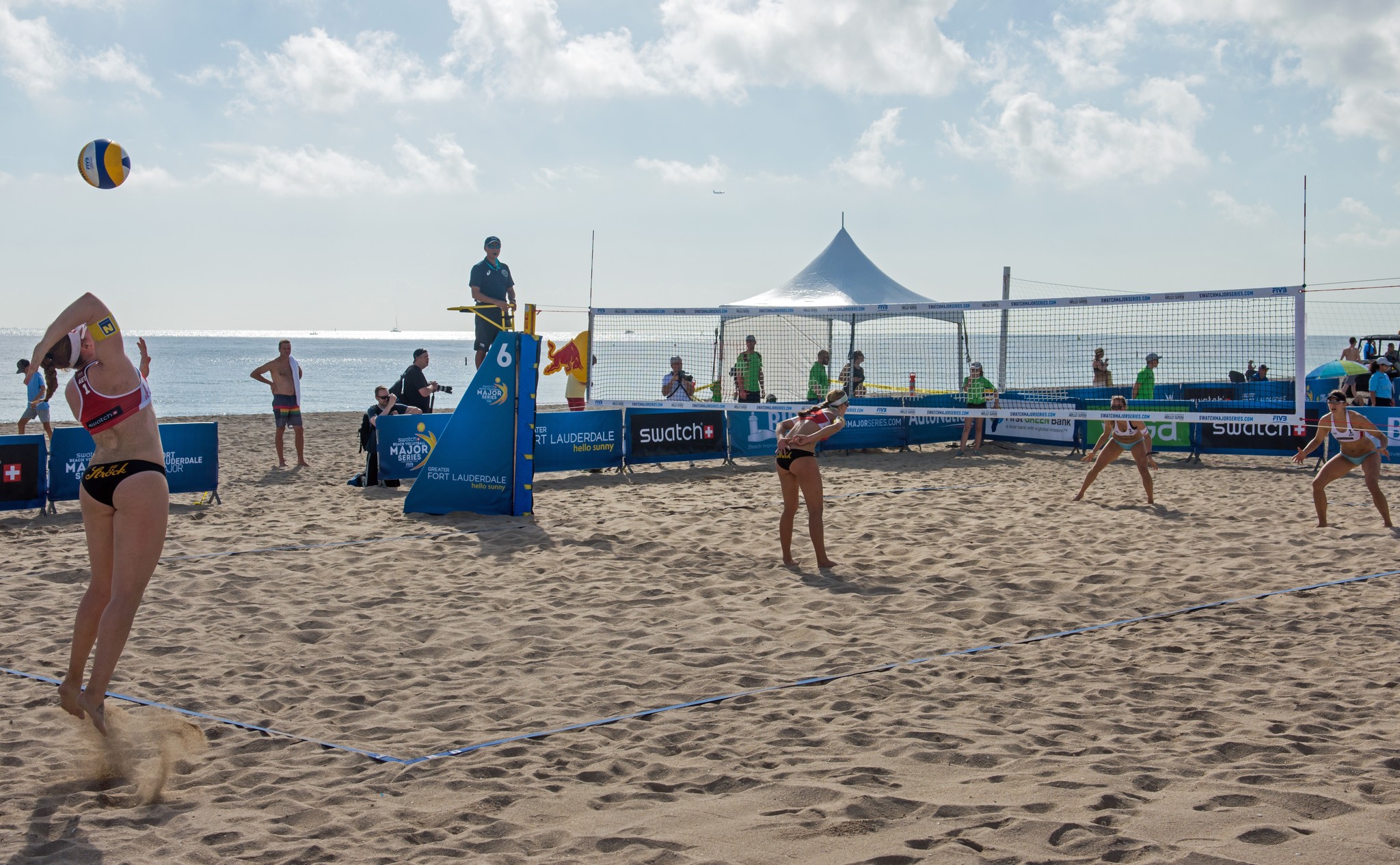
[23,292,170,738]
[367,385,423,452]
[565,355,597,411]
[469,235,517,371]
[736,335,765,403]
[959,337,1400,528]
[808,350,830,401]
[250,339,312,468]
[16,359,53,442]
[847,383,867,398]
[838,350,867,398]
[775,389,849,568]
[661,356,693,402]
[403,348,439,414]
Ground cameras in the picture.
[387,395,398,402]
[676,369,693,382]
[429,381,453,394]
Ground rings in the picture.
[500,307,502,309]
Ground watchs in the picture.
[509,298,516,303]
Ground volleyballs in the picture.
[77,138,131,190]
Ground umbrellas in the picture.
[1306,358,1372,379]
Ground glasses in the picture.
[769,399,776,402]
[1154,359,1158,362]
[1356,341,1357,343]
[487,245,501,249]
[1111,405,1122,410]
[1328,400,1345,405]
[860,392,865,394]
[377,395,389,399]
[822,356,829,359]
[747,341,753,343]
[674,363,683,366]
[1102,353,1104,355]
[1262,369,1268,372]
[845,402,849,407]
[859,355,864,357]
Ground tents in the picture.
[717,228,964,394]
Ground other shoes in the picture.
[1152,452,1159,456]
[689,461,695,467]
[651,463,661,466]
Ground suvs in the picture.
[1358,333,1400,367]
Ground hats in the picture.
[969,361,981,369]
[67,324,84,369]
[670,356,682,365]
[1094,348,1106,356]
[766,394,777,400]
[484,236,501,246]
[1327,394,1346,401]
[1376,357,1393,366]
[747,335,755,341]
[1258,365,1269,371]
[1146,353,1162,362]
[16,359,30,374]
[412,348,427,364]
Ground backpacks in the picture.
[388,366,416,404]
[734,352,761,387]
[358,405,379,451]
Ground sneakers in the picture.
[347,473,361,486]
[955,450,964,457]
[363,470,367,476]
[974,450,981,457]
